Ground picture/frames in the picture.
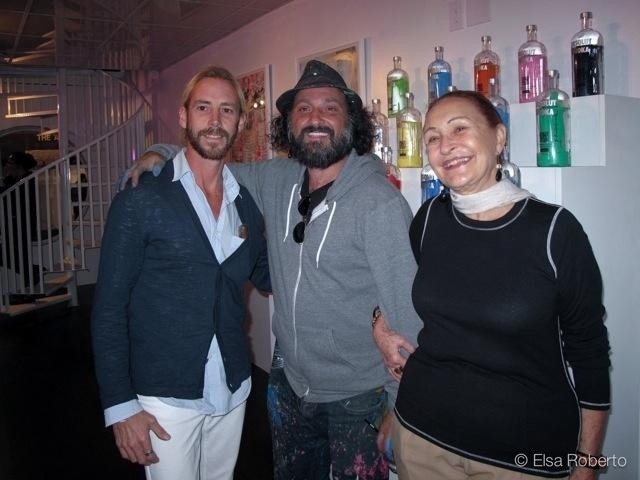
[223,63,275,164]
[294,36,368,111]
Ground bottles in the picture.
[419,165,444,208]
[394,91,425,168]
[427,45,453,111]
[518,25,549,104]
[472,32,502,101]
[368,95,389,166]
[378,144,402,191]
[483,74,511,166]
[385,55,412,120]
[569,10,604,97]
[534,69,572,169]
[501,157,520,189]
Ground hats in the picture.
[276,60,362,115]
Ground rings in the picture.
[143,449,153,456]
[394,366,403,375]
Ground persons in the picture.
[89,66,269,480]
[115,60,424,480]
[371,89,610,480]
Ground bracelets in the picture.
[575,450,608,471]
[372,305,381,326]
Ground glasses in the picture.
[294,197,310,243]
